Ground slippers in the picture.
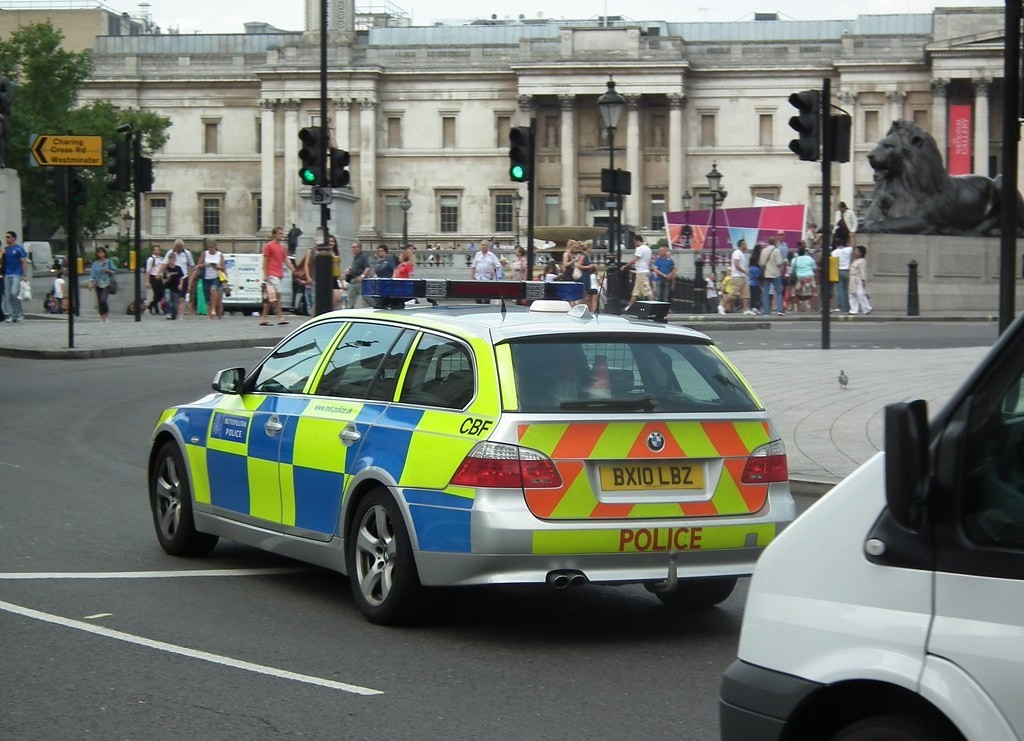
[278,320,288,325]
[260,322,274,325]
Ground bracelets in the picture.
[361,275,366,278]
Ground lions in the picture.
[857,117,1024,242]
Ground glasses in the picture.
[6,236,12,238]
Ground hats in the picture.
[500,257,510,263]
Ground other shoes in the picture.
[16,316,24,322]
[619,308,628,314]
[148,305,153,315]
[752,307,763,314]
[778,311,784,316]
[6,317,16,322]
[156,311,162,315]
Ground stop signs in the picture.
[604,197,619,210]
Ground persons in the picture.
[44,272,65,313]
[706,224,823,316]
[0,231,27,323]
[620,235,677,313]
[259,227,296,325]
[330,235,445,309]
[292,248,314,315]
[144,239,227,320]
[831,201,873,315]
[88,247,117,322]
[511,247,528,281]
[469,237,510,304]
[541,240,602,312]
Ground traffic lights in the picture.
[508,125,533,182]
[331,148,351,187]
[296,125,325,186]
[787,89,823,163]
[138,157,155,192]
[108,141,131,195]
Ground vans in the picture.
[219,254,297,317]
[721,308,1024,741]
[22,240,56,275]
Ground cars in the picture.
[146,279,795,631]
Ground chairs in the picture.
[609,368,635,396]
[336,330,410,399]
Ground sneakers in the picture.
[718,305,725,315]
[743,310,756,315]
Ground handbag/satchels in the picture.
[17,280,32,301]
[572,267,582,280]
[346,272,354,283]
[218,269,229,283]
[789,259,796,285]
[109,279,117,293]
[757,266,765,281]
[829,257,838,282]
[266,281,276,302]
[226,289,230,296]
[61,298,68,309]
[293,257,313,279]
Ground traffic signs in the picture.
[31,134,102,168]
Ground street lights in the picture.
[703,158,724,295]
[399,195,411,248]
[682,189,692,250]
[598,72,626,266]
